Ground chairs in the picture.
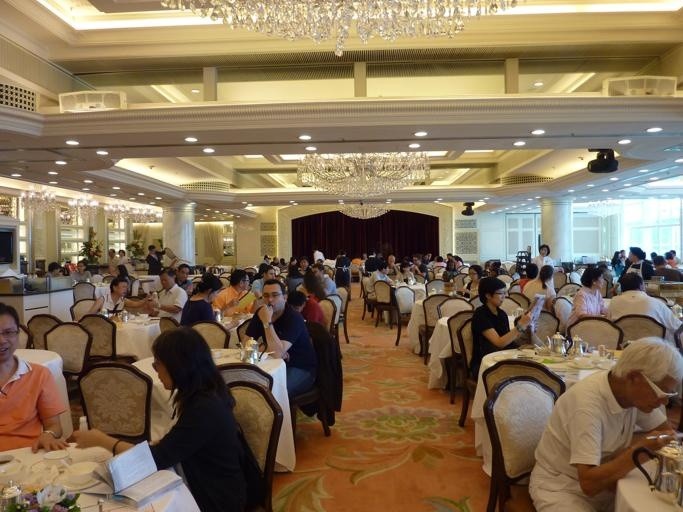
[556,283,582,305]
[128,274,135,282]
[445,310,476,404]
[495,274,514,288]
[76,360,153,445]
[69,299,96,322]
[515,310,560,344]
[243,268,257,276]
[374,280,398,329]
[17,325,33,352]
[275,269,288,292]
[322,265,335,280]
[427,266,447,281]
[77,314,116,360]
[191,276,202,283]
[600,279,608,298]
[336,285,350,344]
[674,324,682,354]
[43,322,92,396]
[214,361,274,393]
[567,317,624,350]
[425,279,446,296]
[482,359,566,497]
[218,277,231,289]
[192,322,231,351]
[361,277,377,321]
[551,296,573,332]
[318,298,337,336]
[554,271,567,293]
[27,313,63,351]
[225,380,284,511]
[101,275,115,285]
[553,266,564,274]
[613,314,667,346]
[453,264,472,288]
[436,297,473,319]
[326,294,343,359]
[507,292,530,309]
[566,269,585,286]
[237,316,253,345]
[508,284,521,293]
[456,318,477,426]
[419,294,451,365]
[483,375,557,512]
[159,317,182,334]
[499,296,522,318]
[72,281,96,303]
[469,295,483,310]
[219,271,231,278]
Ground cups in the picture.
[129,314,135,320]
[671,304,682,319]
[241,349,254,365]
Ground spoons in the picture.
[67,504,81,510]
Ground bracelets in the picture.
[43,429,57,436]
[112,437,123,455]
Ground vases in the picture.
[129,251,134,256]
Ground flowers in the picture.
[10,484,81,512]
[78,238,105,263]
[125,244,139,253]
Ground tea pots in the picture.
[213,310,225,322]
[631,441,683,504]
[234,336,267,364]
[512,306,524,318]
[566,335,589,360]
[547,332,572,358]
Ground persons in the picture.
[0,301,71,452]
[40,244,352,401]
[359,242,683,383]
[72,324,267,512]
[530,338,682,512]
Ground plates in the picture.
[0,457,23,478]
[30,459,73,476]
[567,362,596,369]
[594,365,617,370]
[53,474,103,491]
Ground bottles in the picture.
[122,309,129,324]
[598,344,605,357]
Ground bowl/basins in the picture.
[43,451,70,468]
[66,462,97,484]
[522,349,535,360]
[573,357,591,366]
[597,359,619,367]
[0,454,15,472]
[139,314,148,319]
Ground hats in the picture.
[502,261,516,276]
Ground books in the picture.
[83,441,183,508]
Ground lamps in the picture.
[460,201,474,216]
[20,185,54,213]
[295,151,426,200]
[585,148,618,174]
[336,206,390,220]
[158,0,517,59]
[102,200,130,222]
[66,190,98,219]
[130,206,157,223]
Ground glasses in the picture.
[494,292,505,295]
[264,293,283,297]
[469,272,478,276]
[0,328,19,337]
[642,372,679,400]
